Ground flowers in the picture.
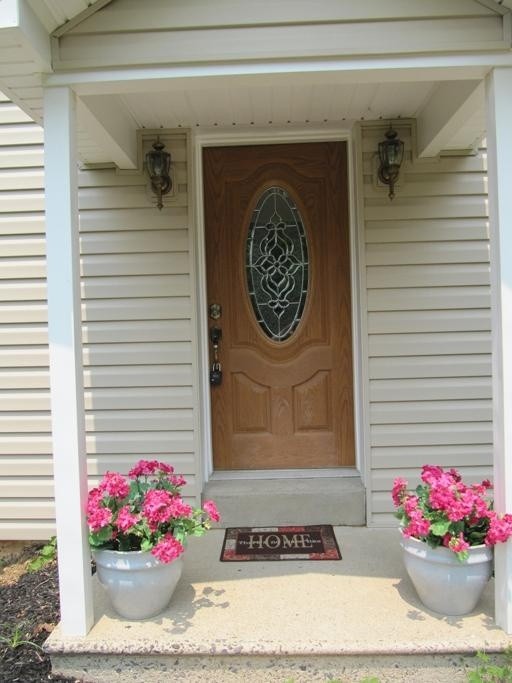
[391,463,512,563]
[31,460,220,570]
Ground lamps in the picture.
[378,122,405,200]
[146,133,172,210]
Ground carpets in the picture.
[219,525,342,562]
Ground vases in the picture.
[90,543,182,620]
[397,524,494,616]
